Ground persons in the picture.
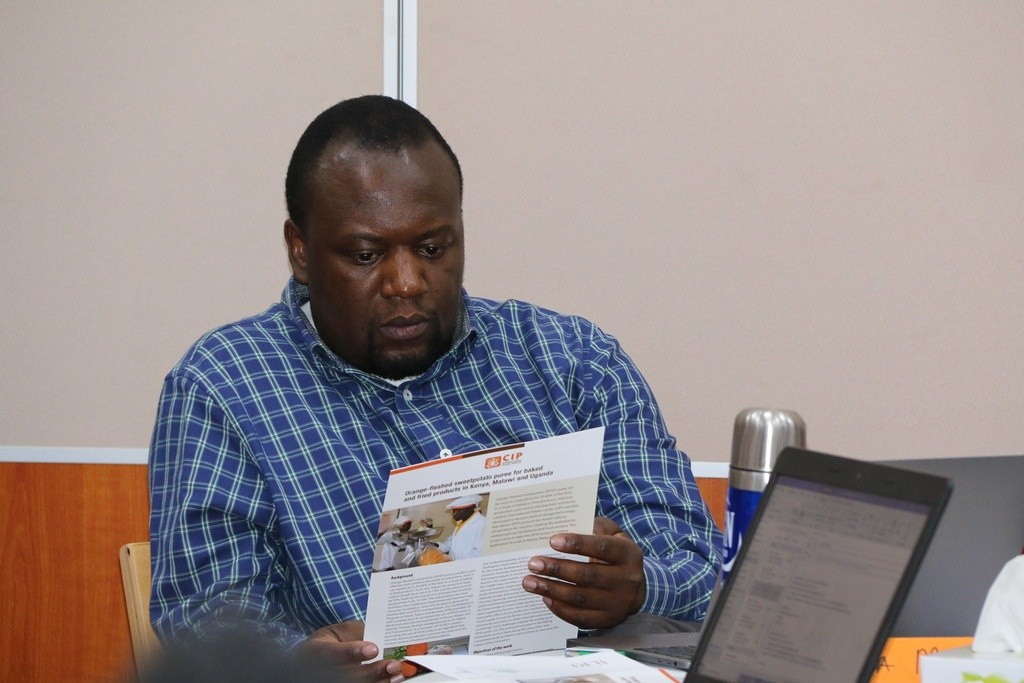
[431,494,486,561]
[377,517,416,571]
[148,94,723,683]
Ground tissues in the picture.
[919,553,1024,683]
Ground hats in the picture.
[447,495,483,510]
[394,515,412,527]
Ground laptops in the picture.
[566,446,955,683]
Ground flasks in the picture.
[721,406,806,588]
